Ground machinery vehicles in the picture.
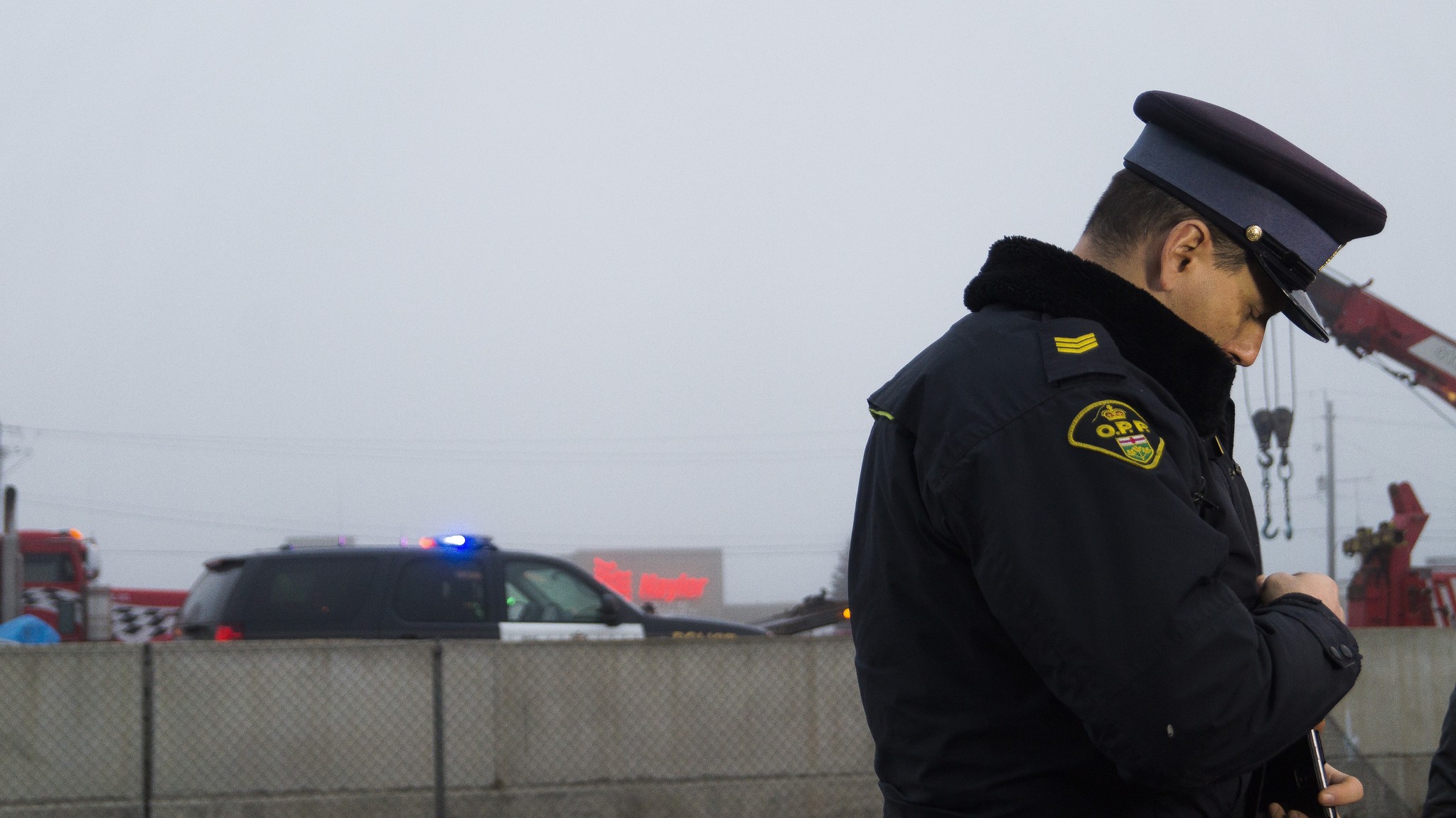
[1241,264,1456,627]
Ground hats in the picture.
[1122,90,1387,344]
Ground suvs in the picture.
[172,537,776,643]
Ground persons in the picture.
[853,88,1389,818]
[1419,680,1456,817]
